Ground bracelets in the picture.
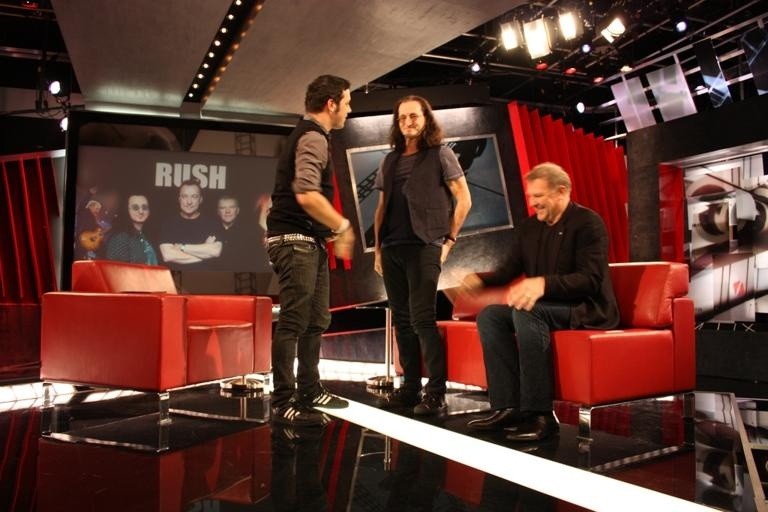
[331,218,350,234]
[444,233,456,244]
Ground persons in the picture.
[96,179,264,271]
[266,74,356,422]
[373,95,473,416]
[457,161,620,442]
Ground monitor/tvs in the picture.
[345,133,514,254]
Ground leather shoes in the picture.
[507,411,559,440]
[468,409,517,429]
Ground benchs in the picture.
[393,262,696,452]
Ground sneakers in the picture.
[414,394,446,413]
[376,386,422,407]
[270,395,323,426]
[301,383,348,408]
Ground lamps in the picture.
[469,3,697,115]
[42,70,63,96]
[57,116,68,132]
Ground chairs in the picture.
[38,426,272,508]
[40,260,272,451]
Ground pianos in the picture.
[693,289,768,397]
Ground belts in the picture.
[267,234,315,244]
